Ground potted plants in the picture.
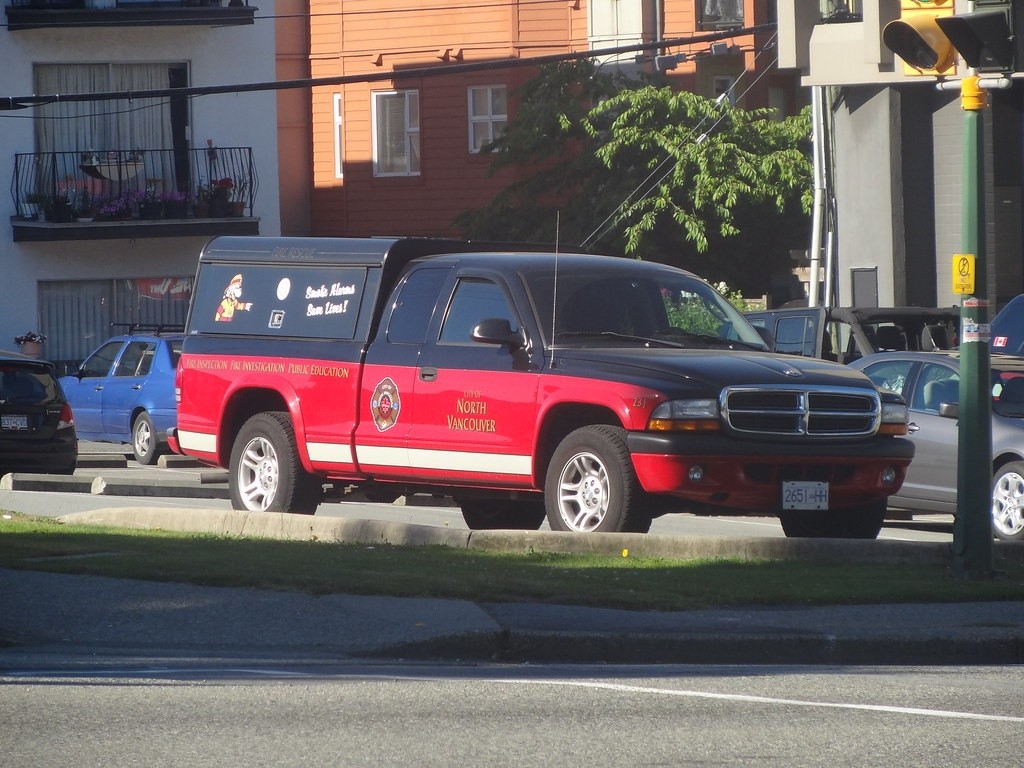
[228,165,252,217]
[20,193,44,218]
[192,176,211,218]
[72,209,95,222]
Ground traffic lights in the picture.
[884,16,951,76]
[933,7,1024,73]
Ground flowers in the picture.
[210,177,233,202]
[96,191,128,215]
[127,186,161,208]
[159,191,195,202]
[12,331,48,348]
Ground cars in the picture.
[720,304,962,366]
[927,291,1022,417]
[58,331,186,465]
[0,348,79,474]
[840,350,1024,545]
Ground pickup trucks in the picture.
[167,235,914,539]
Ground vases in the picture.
[20,342,39,354]
[163,200,188,220]
[138,202,161,220]
[210,202,228,218]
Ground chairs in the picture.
[922,325,948,351]
[846,326,878,365]
[923,379,959,412]
[802,327,839,362]
[876,326,905,351]
[992,377,1024,417]
